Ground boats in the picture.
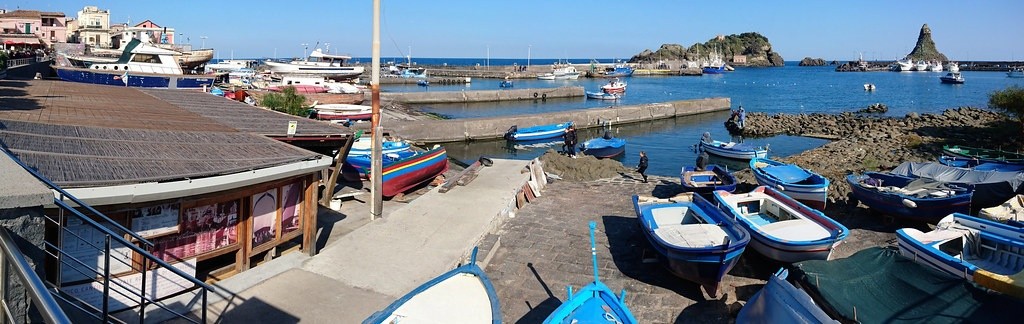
[705,151,751,171]
[503,120,576,142]
[49,36,384,120]
[845,171,975,223]
[585,43,735,78]
[940,70,964,84]
[735,192,1024,324]
[332,136,449,197]
[889,143,1024,211]
[540,220,640,324]
[887,54,959,72]
[585,91,621,100]
[600,77,628,95]
[698,132,770,162]
[360,246,502,324]
[390,46,426,78]
[748,157,830,210]
[711,183,849,267]
[578,130,627,159]
[537,57,580,80]
[417,80,430,86]
[498,76,514,88]
[864,83,876,90]
[680,152,737,201]
[1006,69,1024,78]
[631,192,751,299]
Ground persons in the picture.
[562,125,578,159]
[636,151,648,183]
[732,106,745,127]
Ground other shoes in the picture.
[643,180,648,183]
[645,176,648,179]
[571,155,576,158]
